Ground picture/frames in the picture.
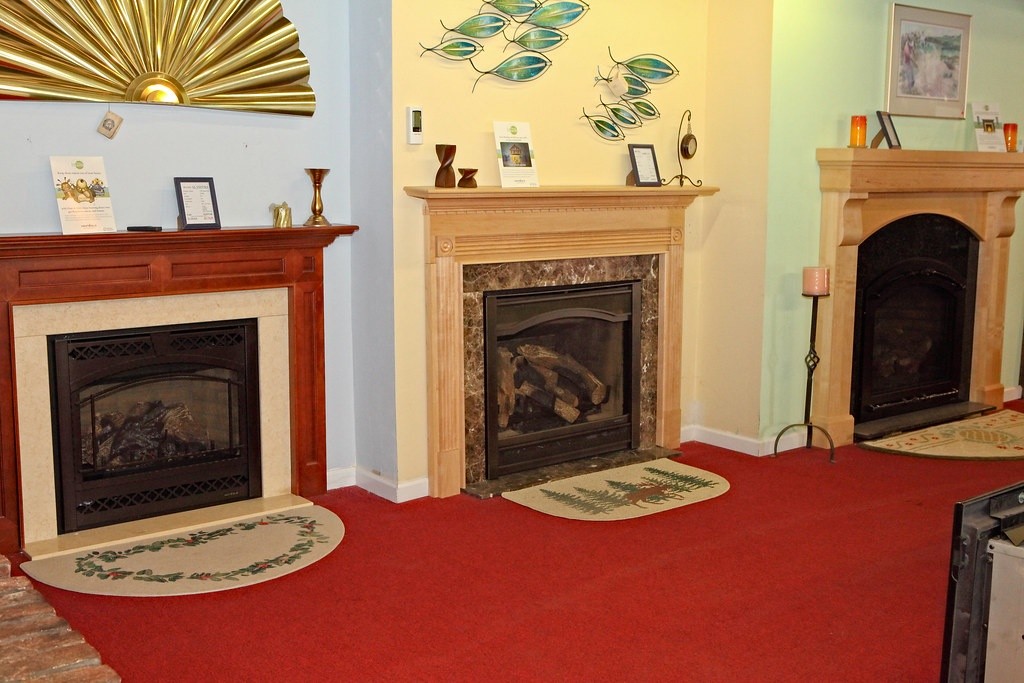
[876,110,901,149]
[174,175,222,230]
[884,2,973,118]
[628,143,661,187]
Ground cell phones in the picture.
[127,226,162,231]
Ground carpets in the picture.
[18,502,345,598]
[499,457,731,521]
[853,406,1024,461]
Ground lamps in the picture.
[773,266,838,463]
